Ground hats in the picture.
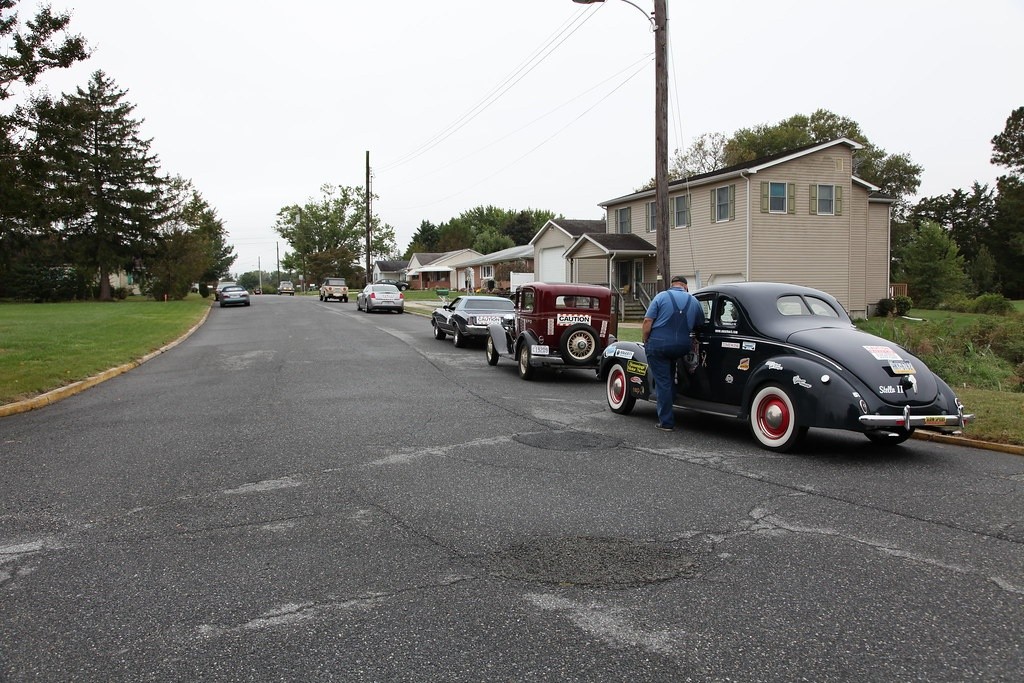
[671,276,688,292]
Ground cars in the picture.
[431,295,521,346]
[278,281,295,296]
[366,279,409,290]
[356,284,405,314]
[220,286,250,307]
[597,282,965,451]
[215,282,237,300]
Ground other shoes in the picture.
[655,423,674,432]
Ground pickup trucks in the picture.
[319,278,349,302]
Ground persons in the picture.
[642,276,705,432]
[563,296,574,308]
[254,285,262,295]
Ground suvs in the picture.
[484,281,614,379]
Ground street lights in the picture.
[561,0,672,322]
